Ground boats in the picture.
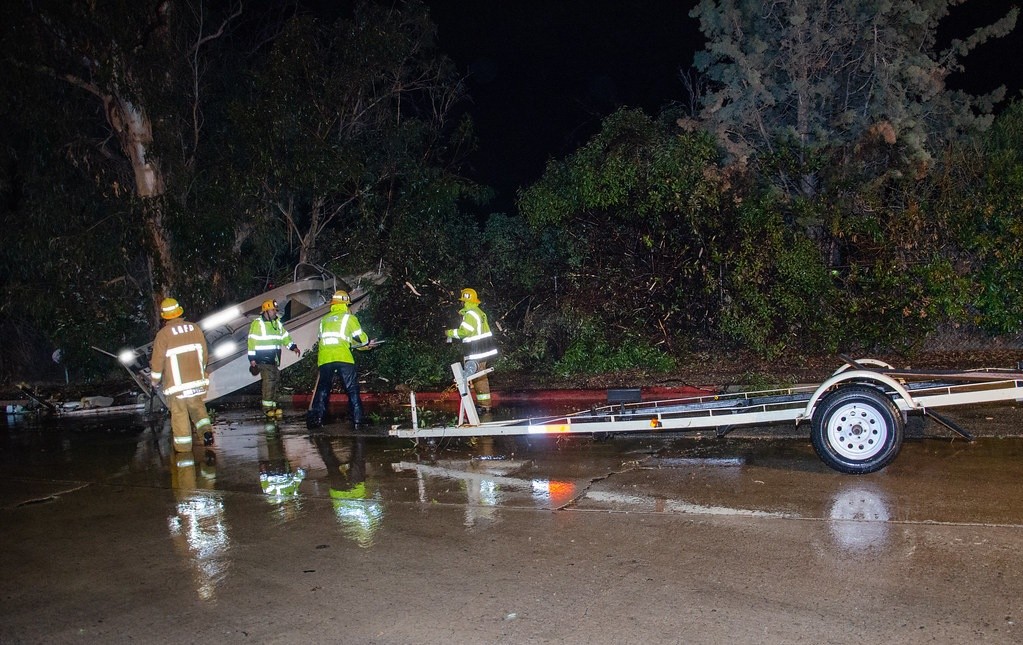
[92,264,390,413]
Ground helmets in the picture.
[331,291,354,304]
[259,301,281,315]
[160,299,184,320]
[459,288,481,304]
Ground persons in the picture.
[246,300,301,418]
[310,432,383,550]
[149,297,215,454]
[256,416,304,524]
[164,455,229,600]
[304,289,374,430]
[444,287,500,414]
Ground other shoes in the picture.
[352,420,369,426]
[266,408,283,416]
[204,432,214,446]
[307,417,323,425]
